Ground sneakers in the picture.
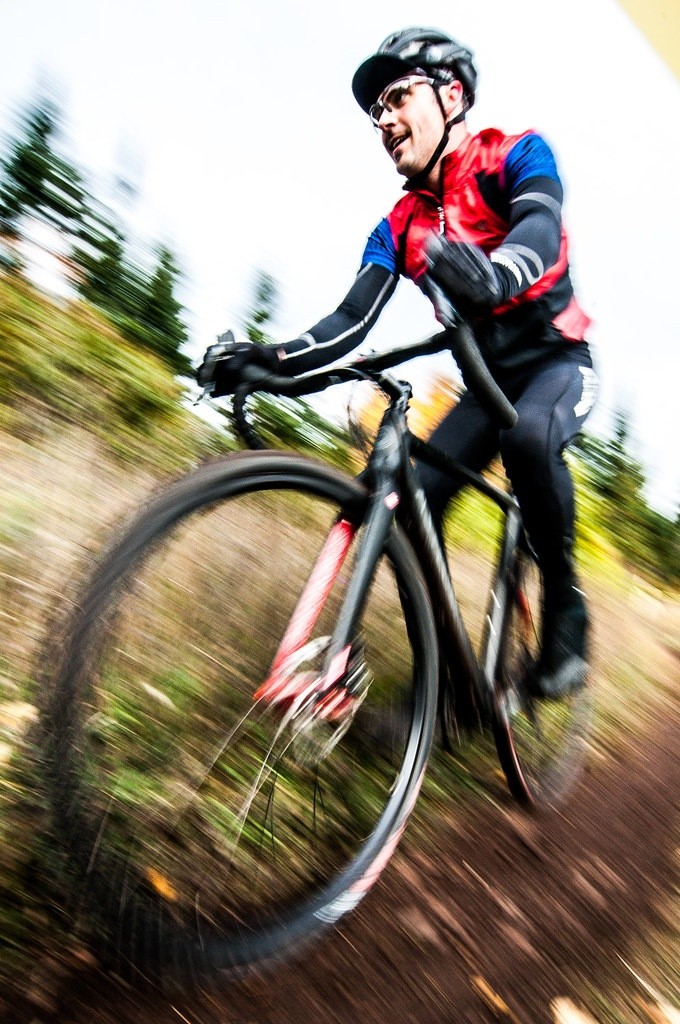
[533,596,592,699]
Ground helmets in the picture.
[352,26,476,108]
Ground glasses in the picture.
[368,75,448,127]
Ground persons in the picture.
[195,28,610,691]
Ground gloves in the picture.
[423,239,510,308]
[194,341,274,402]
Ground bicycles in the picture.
[41,282,596,983]
